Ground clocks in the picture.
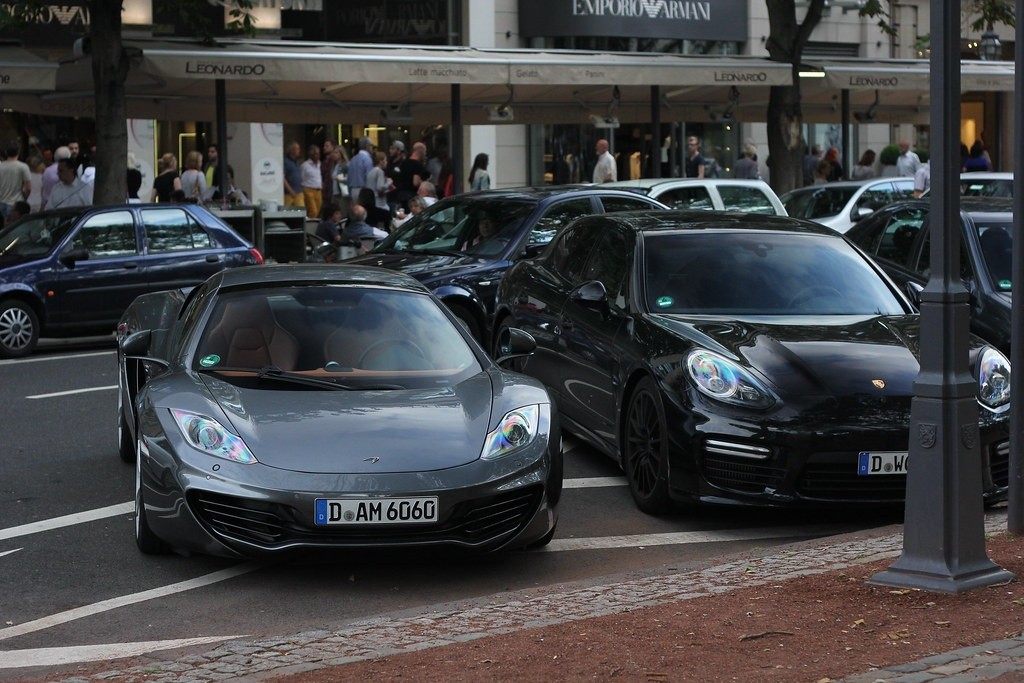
[978,26,1004,62]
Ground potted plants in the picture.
[880,144,901,178]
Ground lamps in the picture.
[485,85,516,120]
[320,82,358,115]
[589,86,625,128]
[661,86,703,122]
[706,86,743,121]
[851,89,881,125]
[381,100,414,122]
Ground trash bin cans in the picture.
[209,204,306,262]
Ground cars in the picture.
[778,178,925,234]
[917,172,1014,201]
[591,178,789,217]
[493,209,1010,515]
[844,196,1013,358]
[335,185,671,360]
[0,203,266,358]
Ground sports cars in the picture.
[117,265,562,562]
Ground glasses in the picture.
[687,143,697,147]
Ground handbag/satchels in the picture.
[193,171,205,207]
[338,173,349,198]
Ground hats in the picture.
[392,141,405,150]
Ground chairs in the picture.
[979,228,1012,282]
[325,294,434,370]
[892,224,920,263]
[207,296,302,371]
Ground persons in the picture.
[686,136,705,180]
[284,135,452,263]
[913,160,930,198]
[464,216,509,250]
[804,143,842,185]
[851,148,875,179]
[467,152,490,190]
[738,138,757,160]
[962,140,994,173]
[733,142,760,180]
[0,136,95,225]
[897,138,922,178]
[128,143,251,205]
[593,139,616,183]
